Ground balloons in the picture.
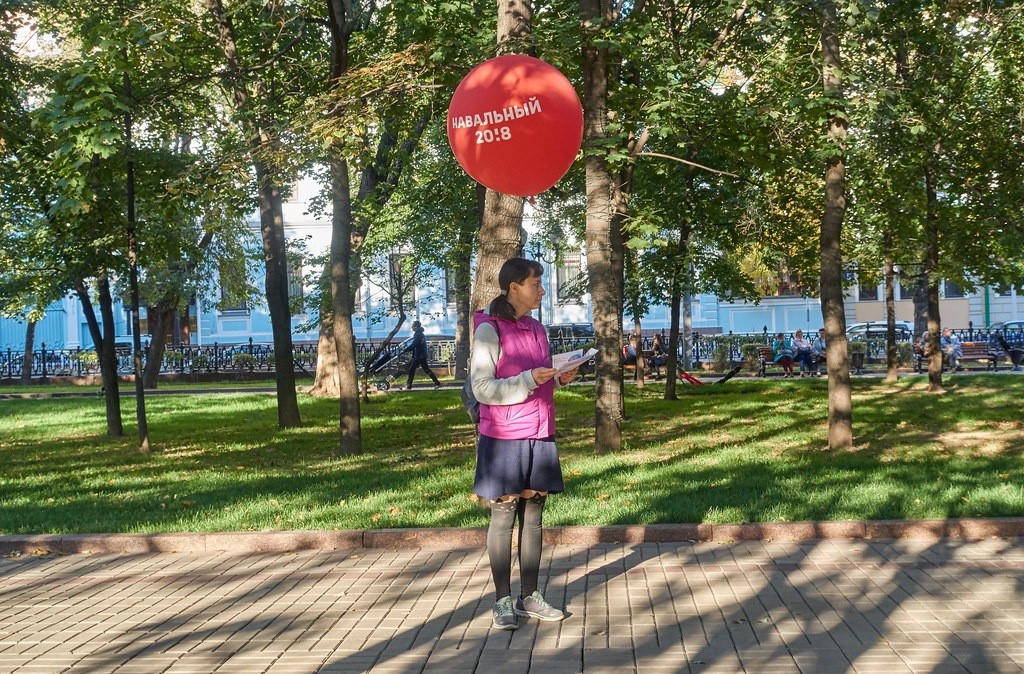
[447,54,584,202]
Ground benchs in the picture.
[912,342,1000,374]
[755,346,818,378]
[621,349,659,379]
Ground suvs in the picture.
[847,321,1024,353]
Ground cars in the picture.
[1,350,67,375]
[211,345,272,360]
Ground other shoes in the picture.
[944,355,949,362]
[951,367,956,373]
[655,375,662,380]
[784,371,822,378]
[401,385,411,390]
[434,383,442,390]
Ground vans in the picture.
[71,334,167,371]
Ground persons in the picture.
[399,320,442,390]
[920,331,929,353]
[941,328,960,372]
[773,333,794,377]
[623,335,649,380]
[814,328,827,376]
[649,333,667,380]
[792,330,814,377]
[470,258,565,630]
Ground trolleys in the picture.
[360,348,410,391]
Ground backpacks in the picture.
[461,320,501,424]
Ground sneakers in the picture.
[516,590,565,622]
[491,595,520,629]
